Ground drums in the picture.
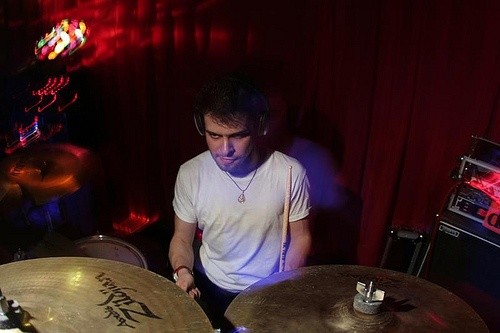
[74,235,148,271]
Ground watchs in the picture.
[172,266,194,281]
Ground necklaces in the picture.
[225,155,259,203]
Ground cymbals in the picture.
[219,265,490,333]
[5,142,99,206]
[0,257,214,333]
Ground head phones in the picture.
[193,75,272,139]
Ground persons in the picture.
[168,70,314,333]
[269,91,336,209]
[0,29,107,235]
